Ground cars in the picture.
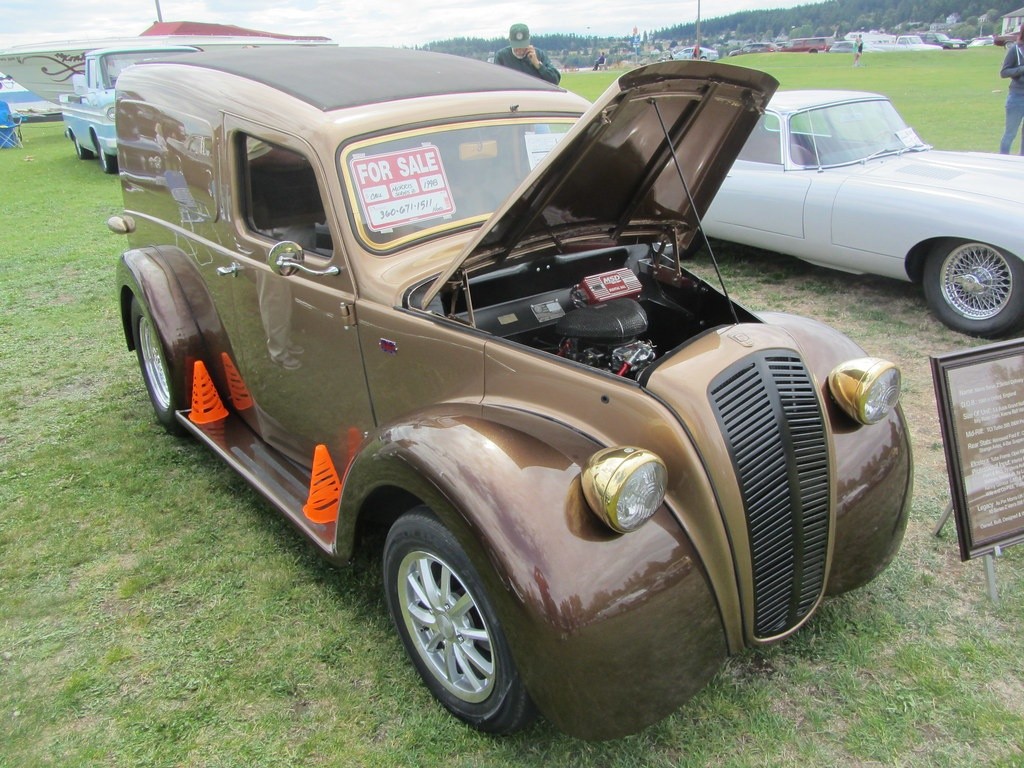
[115,47,915,745]
[671,31,1024,62]
[697,91,1024,339]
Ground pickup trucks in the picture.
[59,48,205,172]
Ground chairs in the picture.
[0,100,24,149]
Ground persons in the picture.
[494,24,561,85]
[1000,21,1024,156]
[853,34,863,66]
[592,51,605,71]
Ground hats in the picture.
[510,24,530,48]
